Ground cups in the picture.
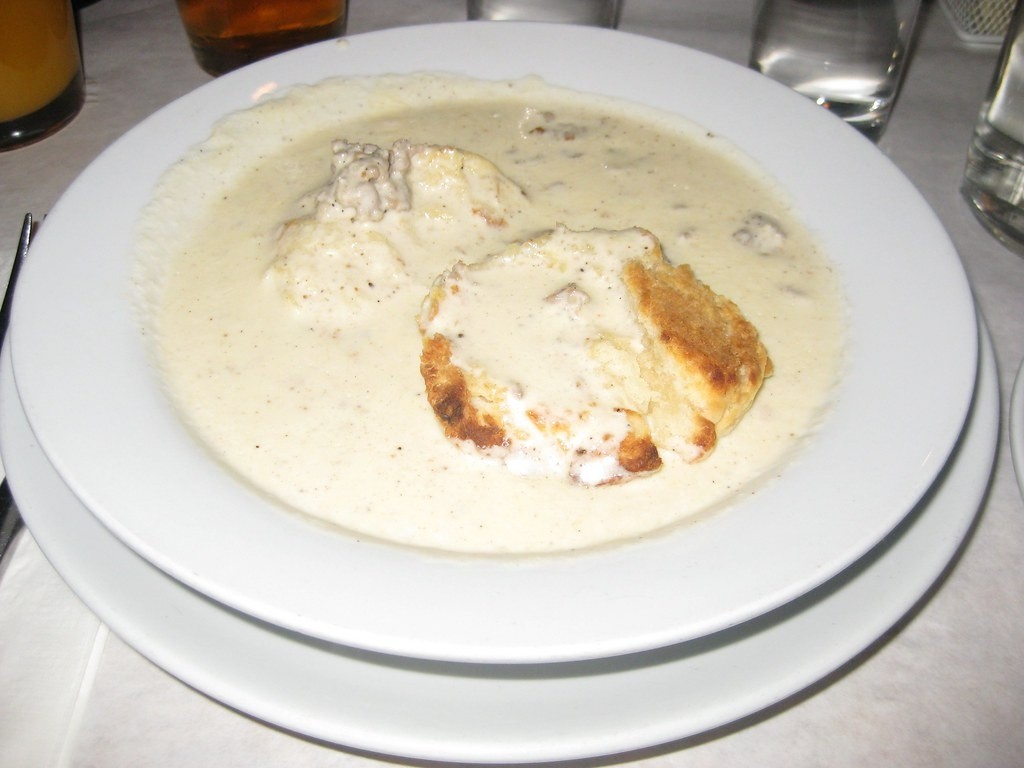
[0,0,88,153]
[961,1,1024,259]
[467,0,620,30]
[747,0,924,144]
[175,0,349,79]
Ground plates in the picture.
[6,22,981,667]
[2,327,1001,766]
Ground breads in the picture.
[414,224,777,492]
